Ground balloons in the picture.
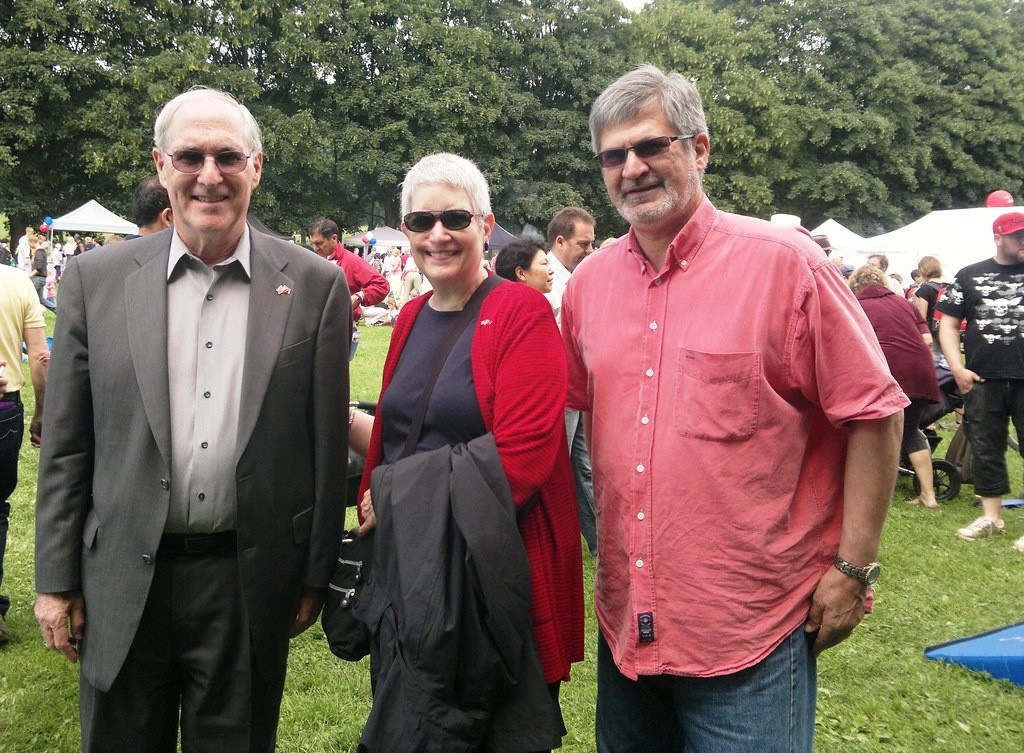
[40,216,52,232]
[362,231,376,245]
[986,190,1014,207]
[491,256,496,265]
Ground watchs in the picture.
[832,555,881,585]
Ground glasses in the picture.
[403,209,481,232]
[161,148,251,174]
[593,134,694,169]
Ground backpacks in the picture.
[927,282,968,336]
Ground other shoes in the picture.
[938,421,960,431]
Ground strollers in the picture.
[894,357,987,505]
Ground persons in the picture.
[0,227,122,315]
[496,207,617,558]
[34,87,353,753]
[0,262,50,642]
[308,218,390,361]
[353,247,435,327]
[933,213,1024,553]
[558,65,909,753]
[350,151,584,753]
[125,175,174,240]
[810,235,966,508]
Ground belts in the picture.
[158,530,238,555]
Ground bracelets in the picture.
[349,405,356,423]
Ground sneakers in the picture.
[954,515,1006,543]
[1011,536,1024,553]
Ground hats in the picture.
[992,212,1024,236]
[812,234,837,251]
[838,264,856,277]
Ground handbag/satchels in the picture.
[320,526,371,662]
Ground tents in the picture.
[489,222,519,246]
[343,226,410,257]
[43,199,139,252]
[807,206,1024,289]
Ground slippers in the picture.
[904,495,940,510]
